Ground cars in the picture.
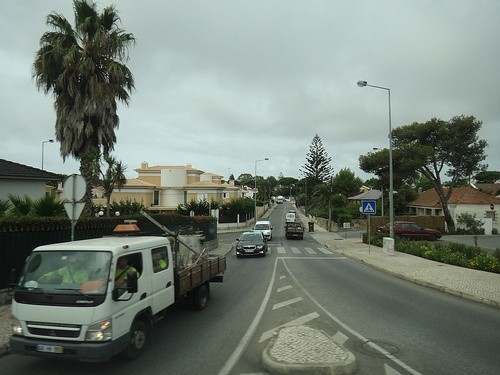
[276,195,285,204]
[376,221,442,241]
[235,232,268,257]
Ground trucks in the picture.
[284,209,303,240]
[9,224,226,363]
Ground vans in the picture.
[252,221,272,241]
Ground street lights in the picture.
[357,80,394,239]
[42,139,54,170]
[255,158,269,221]
[373,147,384,216]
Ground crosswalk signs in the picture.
[361,201,377,214]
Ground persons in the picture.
[103,256,140,290]
[37,254,88,287]
[151,251,167,272]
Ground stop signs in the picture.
[490,204,494,210]
